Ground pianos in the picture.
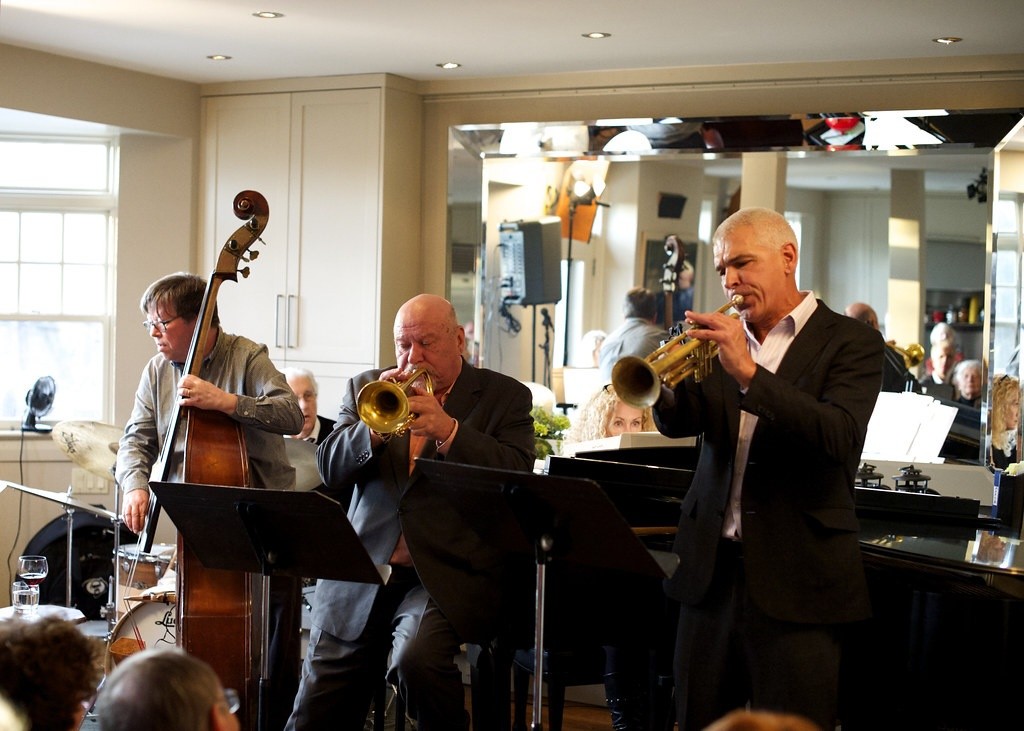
[547,431,1024,731]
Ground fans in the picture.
[23,375,56,433]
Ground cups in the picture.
[12,582,40,615]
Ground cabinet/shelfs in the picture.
[195,87,424,424]
[919,189,991,330]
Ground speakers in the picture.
[496,216,562,308]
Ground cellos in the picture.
[139,184,273,731]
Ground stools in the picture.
[513,642,605,731]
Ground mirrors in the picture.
[445,106,1023,507]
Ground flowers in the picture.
[529,409,572,440]
[529,439,555,461]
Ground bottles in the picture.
[931,294,980,325]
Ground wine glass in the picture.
[18,555,48,620]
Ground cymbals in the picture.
[6,480,120,520]
[283,437,324,492]
[49,419,126,482]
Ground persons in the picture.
[284,295,537,731]
[842,301,1022,473]
[115,273,307,697]
[0,604,242,731]
[573,260,695,443]
[278,365,337,446]
[652,208,886,731]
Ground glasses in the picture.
[224,688,241,716]
[594,383,615,400]
[999,374,1010,386]
[143,316,180,333]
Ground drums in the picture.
[101,591,178,678]
[112,543,178,624]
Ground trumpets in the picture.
[611,293,746,411]
[353,367,434,443]
